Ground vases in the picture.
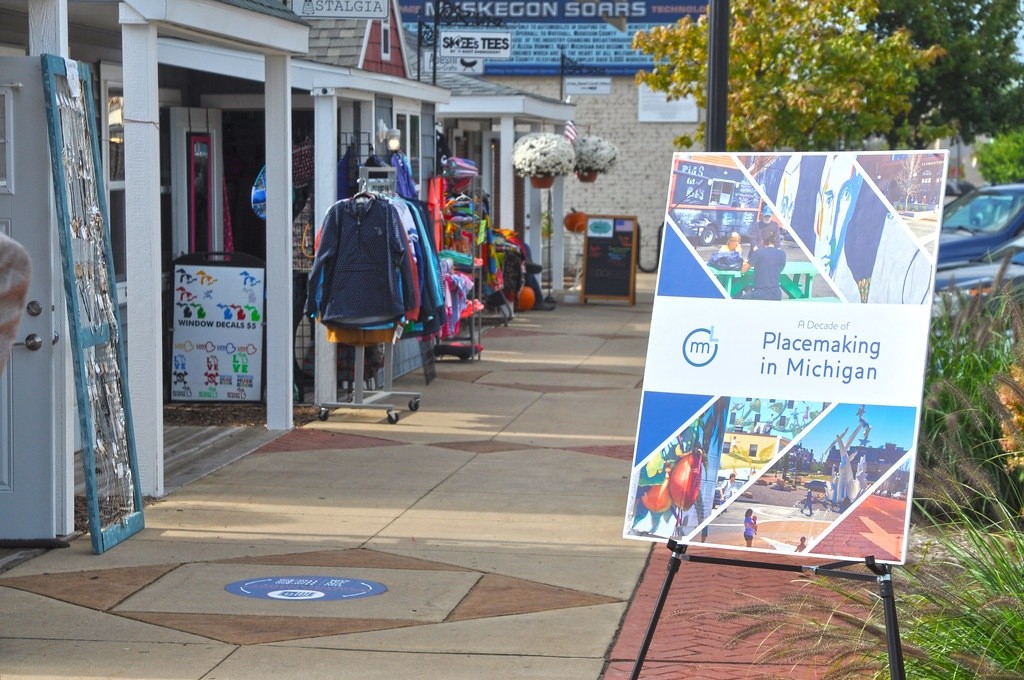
[575,168,598,183]
[529,176,555,188]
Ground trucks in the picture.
[667,158,782,246]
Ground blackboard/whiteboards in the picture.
[582,215,638,300]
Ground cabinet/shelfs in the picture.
[431,174,484,362]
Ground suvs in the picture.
[891,492,906,500]
[874,490,882,496]
[880,490,891,498]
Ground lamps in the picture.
[377,119,400,151]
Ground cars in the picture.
[931,236,1023,315]
[713,479,744,509]
[918,179,1024,267]
[804,480,828,491]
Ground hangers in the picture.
[353,177,398,202]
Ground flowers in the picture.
[570,135,619,176]
[512,132,577,178]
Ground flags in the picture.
[565,98,578,144]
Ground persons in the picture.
[740,229,786,300]
[801,486,813,516]
[0,231,33,376]
[747,206,778,262]
[730,435,742,454]
[794,536,806,552]
[718,232,742,257]
[744,509,757,547]
[701,526,708,542]
[719,474,736,506]
